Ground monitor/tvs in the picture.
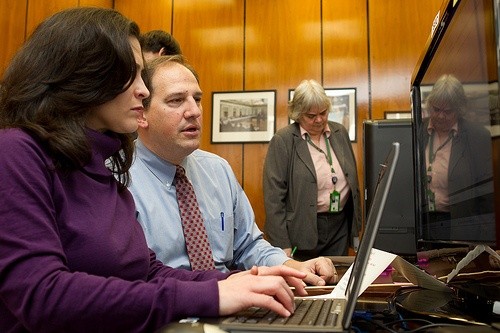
[396,0,500,327]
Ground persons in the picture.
[261,79,362,258]
[0,8,307,333]
[419,73,495,240]
[139,28,183,62]
[104,53,340,286]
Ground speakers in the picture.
[362,119,421,262]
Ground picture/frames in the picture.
[384,111,411,119]
[210,89,277,143]
[288,88,357,143]
[420,80,500,139]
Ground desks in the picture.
[154,260,500,333]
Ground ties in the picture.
[172,164,216,271]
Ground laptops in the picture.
[219,142,400,331]
